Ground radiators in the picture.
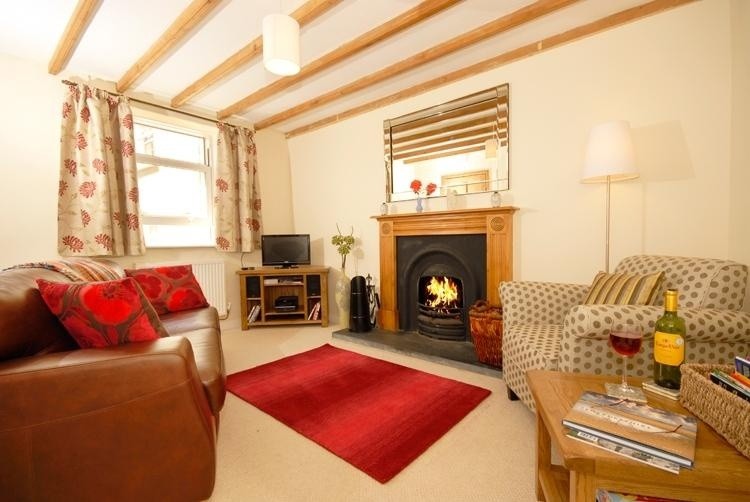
[135,261,231,320]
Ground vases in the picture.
[416,198,424,214]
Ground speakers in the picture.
[307,274,321,296]
[246,276,260,297]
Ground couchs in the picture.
[0,256,226,502]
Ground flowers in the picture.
[410,179,422,199]
[426,183,437,196]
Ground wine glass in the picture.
[611,321,645,400]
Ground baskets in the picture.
[469,299,503,368]
[679,364,750,460]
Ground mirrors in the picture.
[383,83,508,203]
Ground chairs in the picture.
[498,254,750,416]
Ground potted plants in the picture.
[332,223,356,330]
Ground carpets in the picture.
[224,343,492,485]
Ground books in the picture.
[249,305,261,323]
[312,301,321,320]
[247,305,256,320]
[709,355,750,404]
[307,304,317,320]
[562,389,698,469]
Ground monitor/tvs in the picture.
[261,233,311,266]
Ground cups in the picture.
[446,190,459,210]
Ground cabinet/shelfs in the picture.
[526,370,750,502]
[235,265,330,329]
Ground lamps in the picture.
[263,0,301,77]
[580,120,641,272]
[486,123,500,160]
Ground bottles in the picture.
[490,191,502,208]
[379,202,389,215]
[652,288,688,391]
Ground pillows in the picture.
[36,278,170,350]
[582,270,665,305]
[124,263,210,316]
